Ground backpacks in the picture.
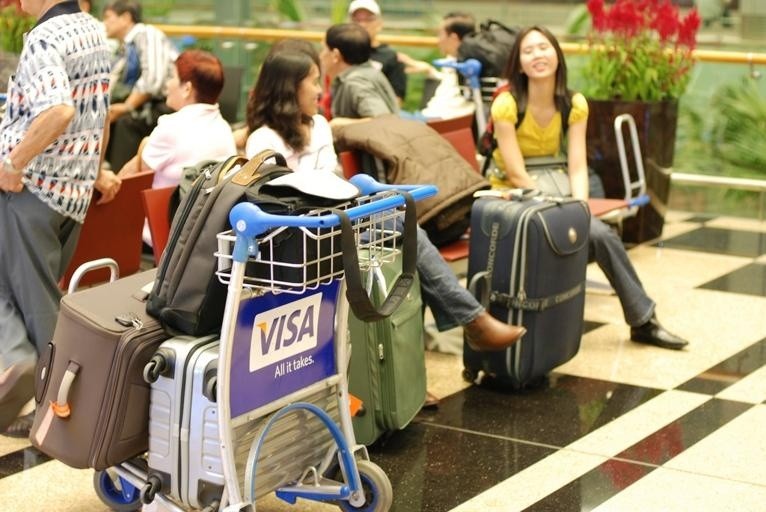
[479,83,573,156]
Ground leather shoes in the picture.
[463,311,525,352]
[630,314,687,349]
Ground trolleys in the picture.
[102,175,440,511]
[589,114,650,248]
[433,51,509,153]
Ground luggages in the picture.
[29,267,160,471]
[140,334,224,512]
[462,187,590,391]
[347,250,426,446]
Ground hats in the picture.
[348,0,380,15]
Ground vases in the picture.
[587,101,680,242]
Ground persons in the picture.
[488,25,687,350]
[245,0,526,351]
[1,1,121,432]
[100,0,235,255]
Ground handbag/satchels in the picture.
[146,148,361,337]
[457,18,522,94]
[523,157,570,196]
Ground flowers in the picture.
[582,1,701,101]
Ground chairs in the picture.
[58,111,652,403]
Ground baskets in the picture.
[213,189,407,295]
